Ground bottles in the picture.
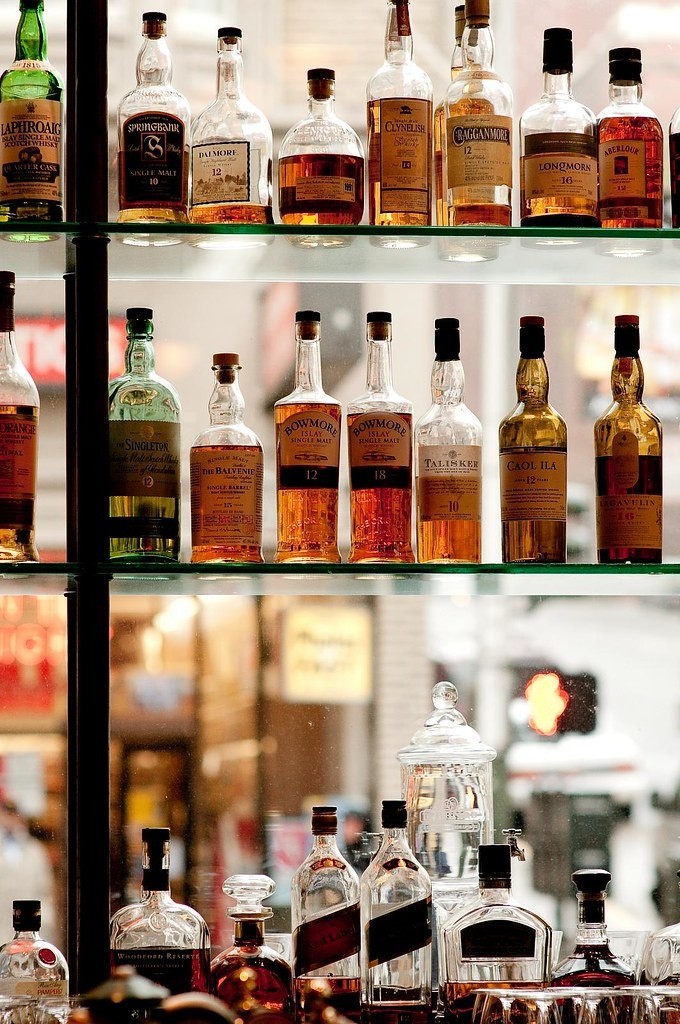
[279,68,364,249]
[188,27,275,253]
[273,310,342,563]
[105,307,181,569]
[188,352,265,566]
[345,312,414,565]
[366,0,680,260]
[116,12,192,246]
[498,315,567,561]
[0,267,40,562]
[592,313,665,564]
[0,0,66,246]
[417,319,483,565]
[0,681,680,1022]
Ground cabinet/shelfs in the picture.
[0,0,680,1024]
